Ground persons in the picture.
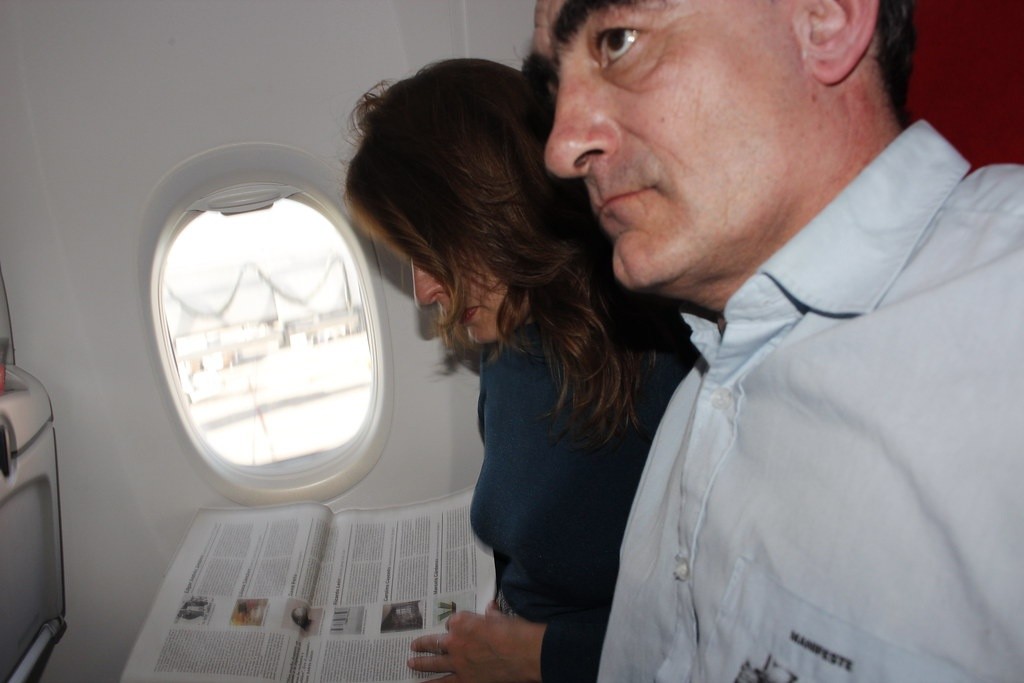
[344,58,720,683]
[523,0,1023,683]
